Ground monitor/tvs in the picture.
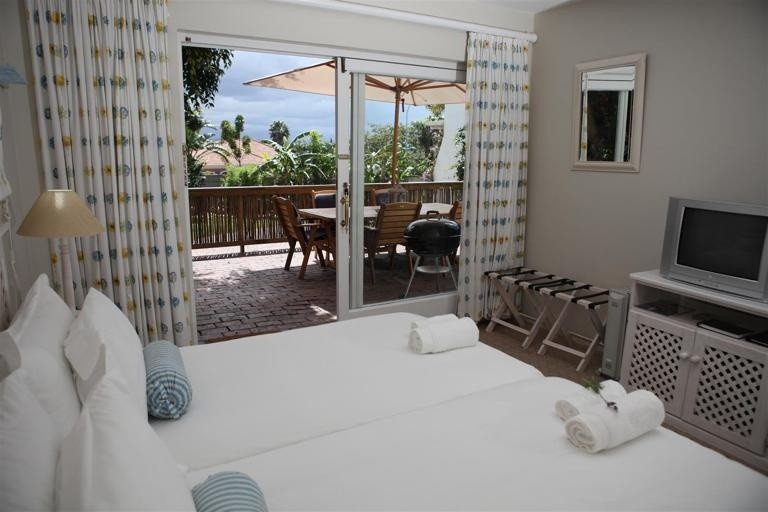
[660,196,767,303]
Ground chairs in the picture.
[269,187,465,300]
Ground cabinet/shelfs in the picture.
[617,266,766,475]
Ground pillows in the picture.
[0,274,75,393]
[64,288,147,423]
[52,374,198,512]
[191,471,266,512]
[143,340,192,419]
[0,364,77,510]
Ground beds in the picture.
[144,308,767,512]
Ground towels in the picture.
[410,313,457,329]
[554,379,627,423]
[564,388,666,454]
[408,317,480,355]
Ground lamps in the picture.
[16,189,104,316]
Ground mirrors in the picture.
[568,53,646,174]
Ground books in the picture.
[697,318,755,343]
[747,330,768,348]
[669,306,704,327]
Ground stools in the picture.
[536,278,609,373]
[483,266,559,351]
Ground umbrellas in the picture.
[242,61,467,204]
